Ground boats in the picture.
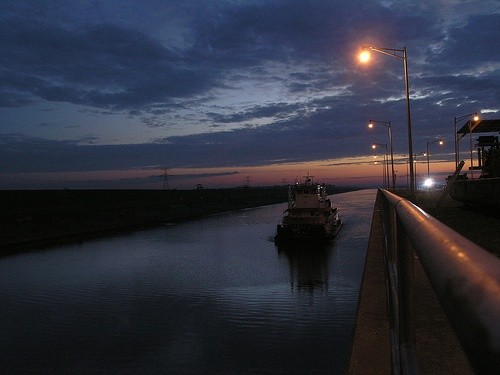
[272,171,342,243]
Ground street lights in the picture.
[374,153,387,191]
[371,142,390,192]
[454,112,480,174]
[426,138,443,180]
[374,161,384,188]
[407,159,416,191]
[414,149,426,190]
[357,43,416,205]
[366,118,396,194]
